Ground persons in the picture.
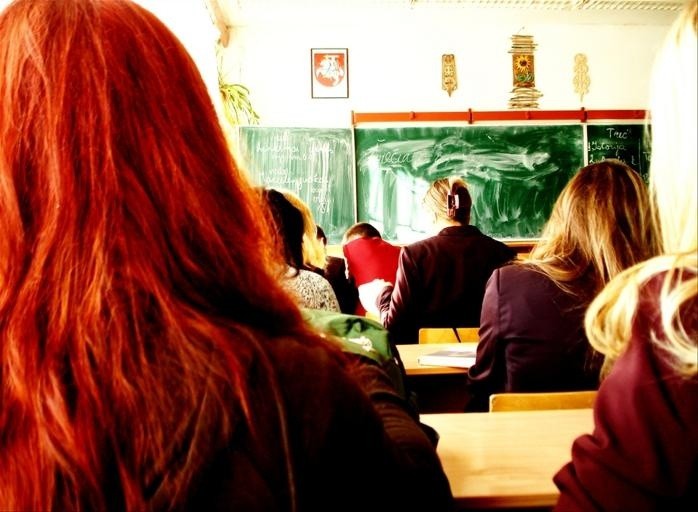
[548,3,696,512]
[374,173,520,356]
[339,219,403,325]
[0,2,459,512]
[303,223,359,316]
[246,182,345,316]
[461,153,665,413]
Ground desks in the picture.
[395,339,597,512]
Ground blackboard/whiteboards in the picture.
[239,125,354,247]
[588,125,651,185]
[354,125,584,241]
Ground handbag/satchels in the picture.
[301,306,396,373]
